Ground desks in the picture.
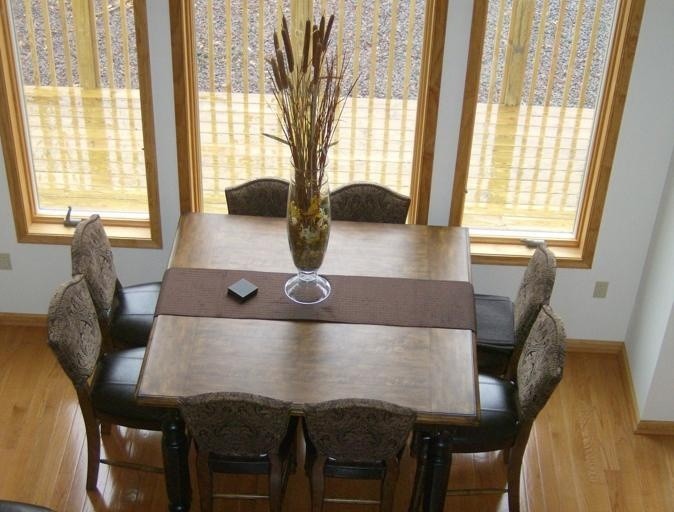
[134,213,481,512]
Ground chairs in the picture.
[175,392,298,512]
[225,176,290,217]
[301,398,417,512]
[47,274,200,498]
[71,214,162,353]
[408,304,567,512]
[329,181,411,224]
[474,242,557,381]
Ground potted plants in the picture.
[261,0,362,305]
